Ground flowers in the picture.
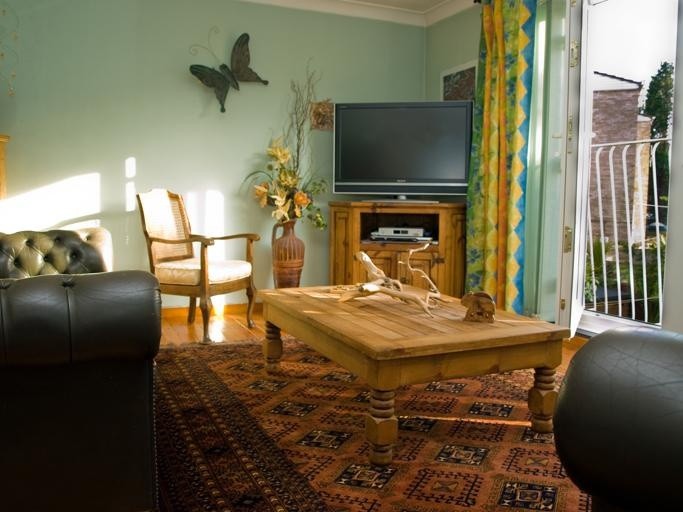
[237,55,329,231]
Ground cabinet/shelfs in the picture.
[328,201,466,298]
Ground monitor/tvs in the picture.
[333,99,472,205]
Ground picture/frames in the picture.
[440,59,478,117]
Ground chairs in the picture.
[136,188,261,345]
[553,328,683,512]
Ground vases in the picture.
[271,220,305,288]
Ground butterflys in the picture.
[188,25,269,114]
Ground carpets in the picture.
[152,337,592,512]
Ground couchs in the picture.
[1,226,163,512]
